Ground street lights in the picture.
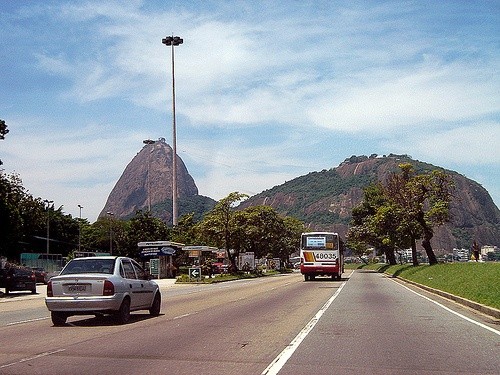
[107,212,115,256]
[78,205,83,251]
[162,35,184,226]
[44,200,54,253]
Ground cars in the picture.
[271,257,301,270]
[45,255,161,327]
[343,247,469,263]
[0,264,60,294]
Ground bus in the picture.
[299,231,347,281]
